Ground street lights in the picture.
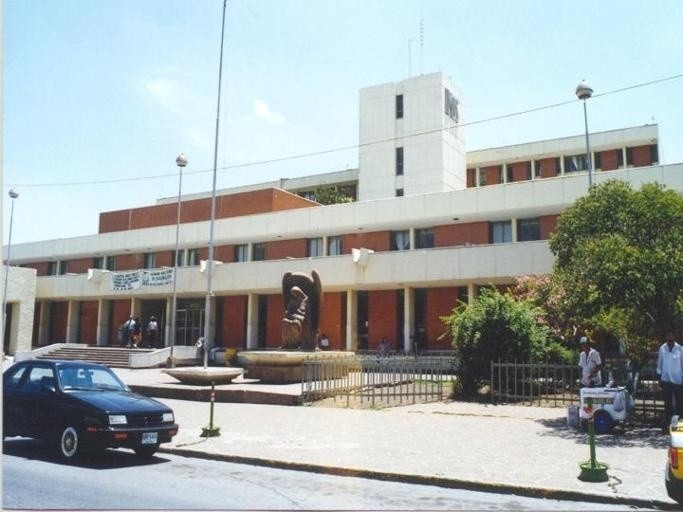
[575,79,593,187]
[1,184,20,363]
[166,151,189,369]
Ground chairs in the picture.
[42,376,88,389]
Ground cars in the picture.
[3,359,179,460]
[665,415,683,507]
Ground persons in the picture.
[320,334,330,349]
[577,336,604,388]
[279,286,309,349]
[379,337,392,358]
[117,312,158,348]
[656,330,683,424]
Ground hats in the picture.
[579,336,589,344]
